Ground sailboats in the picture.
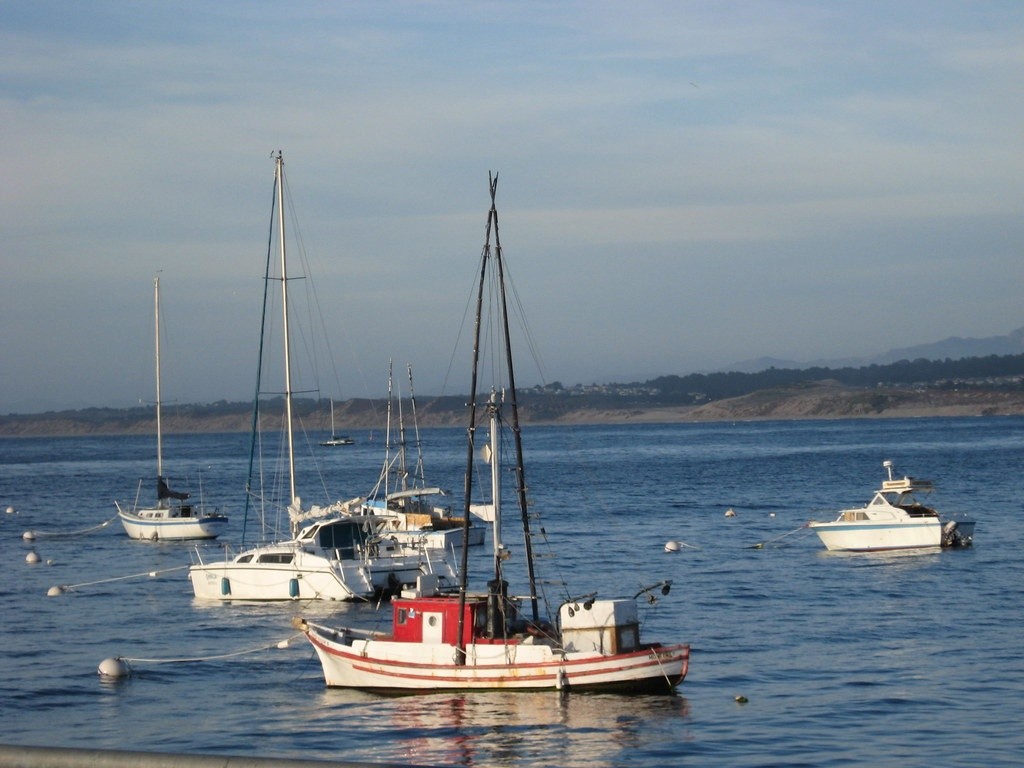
[319,395,355,446]
[185,150,435,612]
[312,349,490,554]
[109,277,234,546]
[285,169,695,700]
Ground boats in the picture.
[805,463,978,552]
